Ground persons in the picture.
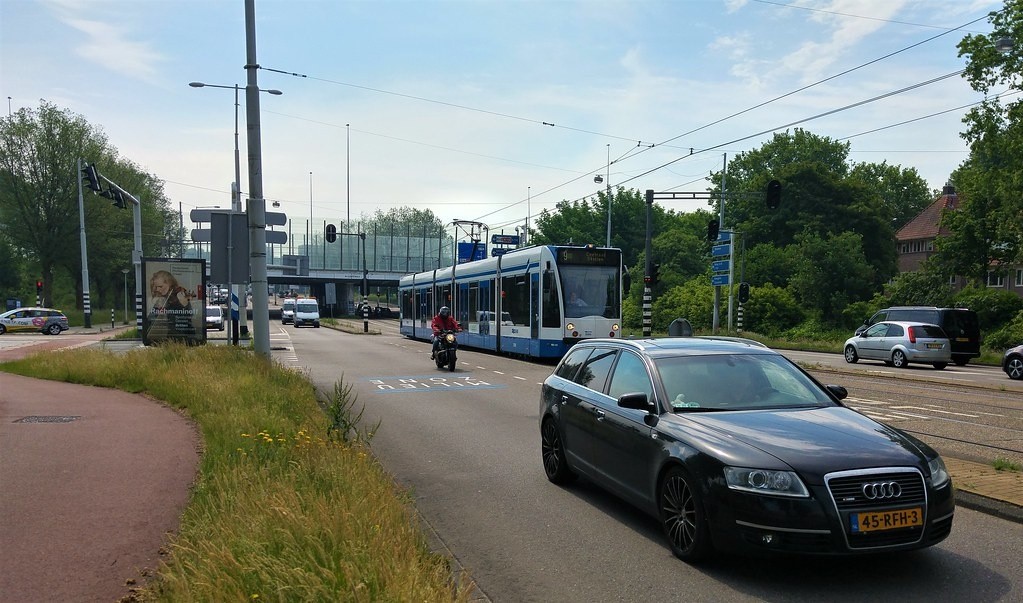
[150,270,196,337]
[567,292,588,307]
[430,305,462,360]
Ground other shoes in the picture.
[431,354,434,360]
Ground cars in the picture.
[842,320,952,370]
[1001,344,1023,380]
[0,306,70,336]
[357,303,391,318]
[538,334,956,569]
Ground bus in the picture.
[397,235,623,362]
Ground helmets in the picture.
[440,307,450,317]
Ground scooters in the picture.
[430,323,464,372]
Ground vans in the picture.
[293,296,320,328]
[476,311,515,327]
[852,305,981,366]
[280,297,296,324]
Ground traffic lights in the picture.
[707,219,719,246]
[80,162,103,192]
[100,190,116,201]
[111,188,127,209]
[649,260,662,286]
[36,280,44,289]
[765,179,783,211]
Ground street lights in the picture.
[8,96,12,178]
[346,123,350,229]
[528,186,531,245]
[309,171,313,268]
[189,82,284,347]
